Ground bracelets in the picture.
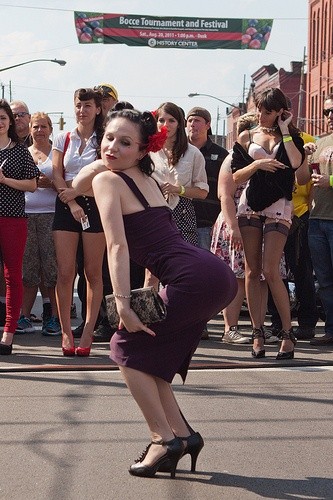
[0,176,5,183]
[329,175,333,187]
[177,186,186,197]
[113,291,132,300]
[281,133,290,136]
[283,137,292,142]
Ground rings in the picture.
[237,241,241,244]
[305,146,310,150]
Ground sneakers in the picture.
[262,327,278,342]
[15,311,62,336]
[222,325,250,343]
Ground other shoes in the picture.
[270,325,282,335]
[201,327,208,339]
[311,334,333,346]
[92,330,110,342]
[294,328,315,340]
[71,321,86,338]
[71,304,77,318]
[0,302,6,330]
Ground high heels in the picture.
[0,341,12,355]
[173,426,204,472]
[127,435,183,479]
[62,336,75,357]
[77,340,93,355]
[252,327,265,359]
[275,327,297,360]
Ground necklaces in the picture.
[0,138,11,151]
[38,155,41,160]
[259,125,278,133]
[24,133,30,141]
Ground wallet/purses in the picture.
[105,286,167,328]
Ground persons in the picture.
[0,84,333,361]
[90,109,238,480]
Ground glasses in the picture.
[12,112,29,118]
[323,108,333,116]
[93,85,117,98]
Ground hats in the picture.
[187,107,213,136]
[99,84,119,102]
